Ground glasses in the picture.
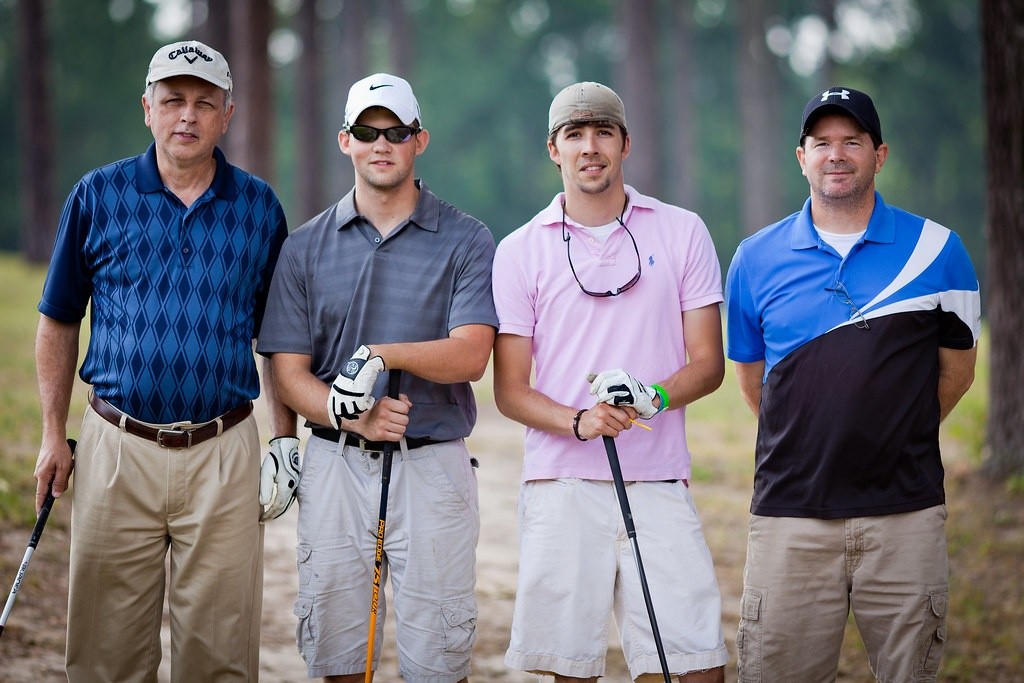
[341,123,424,144]
[564,217,641,299]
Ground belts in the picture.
[88,387,259,451]
[311,424,455,452]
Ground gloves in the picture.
[257,435,301,524]
[586,369,669,421]
[327,343,385,430]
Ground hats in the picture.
[799,85,883,150]
[146,40,233,94]
[547,82,628,134]
[345,73,425,131]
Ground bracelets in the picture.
[652,385,672,413]
[574,409,590,442]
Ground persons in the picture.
[724,86,988,683]
[494,81,734,683]
[252,72,503,683]
[35,37,306,681]
